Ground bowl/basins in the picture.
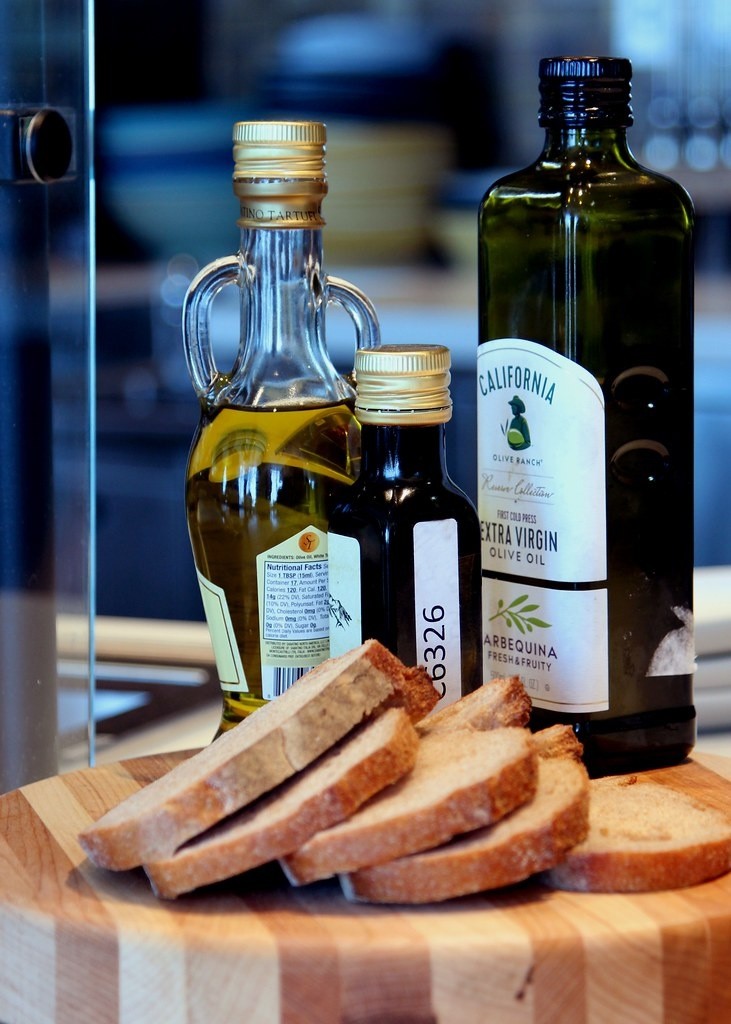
[104,107,521,268]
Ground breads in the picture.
[76,638,731,906]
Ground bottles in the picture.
[188,118,380,747]
[324,345,482,719]
[479,54,697,770]
